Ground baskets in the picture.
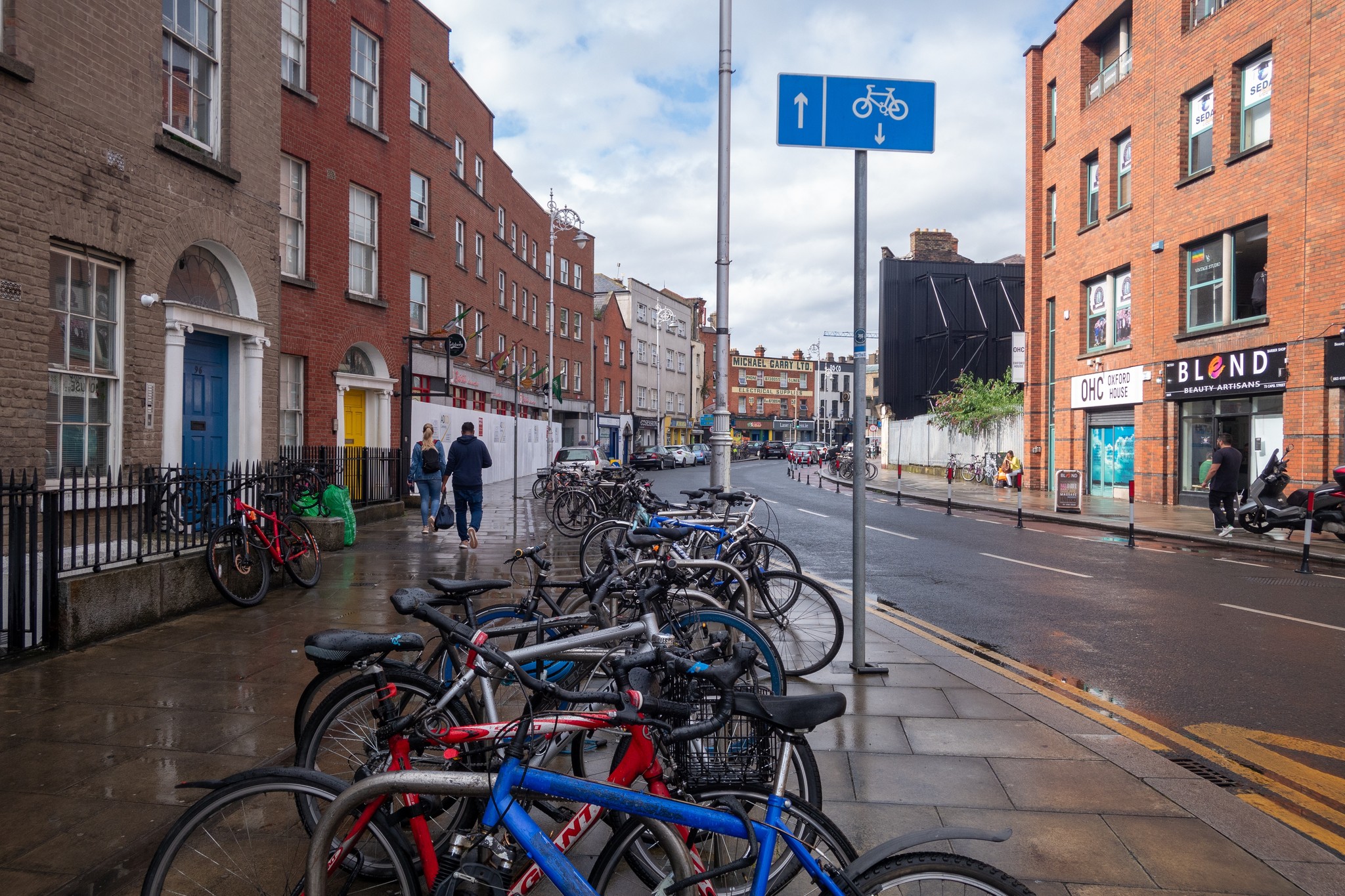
[662,668,781,788]
[536,468,551,477]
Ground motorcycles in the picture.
[1238,443,1345,543]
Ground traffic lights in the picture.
[796,420,799,428]
[793,420,795,429]
[729,424,732,431]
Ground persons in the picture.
[1117,311,1131,341]
[594,440,600,447]
[1002,450,1021,489]
[407,423,446,533]
[441,422,492,548]
[1093,316,1106,347]
[706,440,711,448]
[1199,452,1213,487]
[578,435,588,446]
[1252,263,1267,316]
[1201,433,1243,538]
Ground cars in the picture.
[743,440,837,464]
[629,445,676,470]
[663,444,697,468]
[686,444,712,465]
[554,445,611,480]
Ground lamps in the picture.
[1156,376,1163,385]
[1277,367,1289,378]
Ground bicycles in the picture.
[828,452,878,480]
[140,460,1034,896]
[944,453,961,479]
[204,473,322,609]
[961,455,985,483]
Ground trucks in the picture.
[846,436,881,454]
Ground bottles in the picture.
[408,479,414,492]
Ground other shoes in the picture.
[1240,502,1245,506]
[1004,485,1013,488]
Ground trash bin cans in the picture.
[985,452,1007,487]
[875,446,879,455]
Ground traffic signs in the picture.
[700,414,714,426]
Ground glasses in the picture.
[598,442,599,443]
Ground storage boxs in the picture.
[1333,463,1345,487]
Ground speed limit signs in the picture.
[709,426,714,433]
[869,424,876,432]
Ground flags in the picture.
[492,344,513,371]
[521,367,547,389]
[434,310,471,334]
[509,364,531,384]
[552,375,563,404]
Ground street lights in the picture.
[547,188,590,468]
[655,297,679,445]
[824,362,835,442]
[805,335,820,442]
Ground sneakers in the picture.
[458,540,468,548]
[427,516,438,532]
[1224,532,1233,538]
[468,527,478,549]
[421,526,429,534]
[1218,525,1234,537]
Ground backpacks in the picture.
[416,440,442,474]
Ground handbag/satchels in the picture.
[993,474,1004,488]
[998,472,1007,481]
[1002,460,1011,474]
[433,490,454,532]
